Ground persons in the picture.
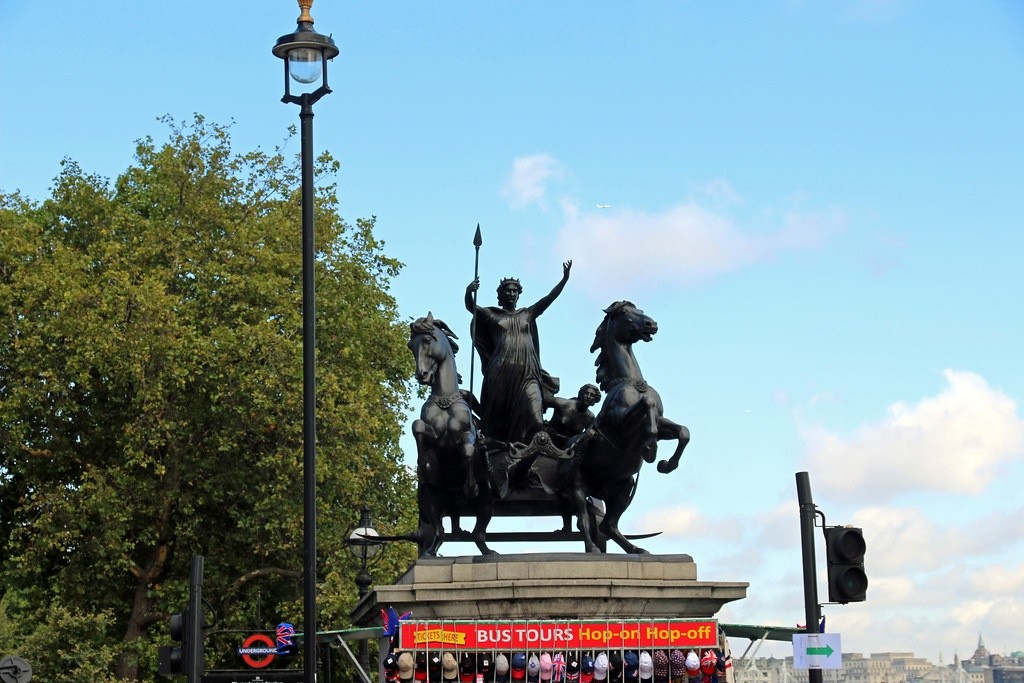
[465,260,602,445]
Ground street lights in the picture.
[344,508,387,683]
[272,0,339,683]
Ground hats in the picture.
[384,649,726,683]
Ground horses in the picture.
[406,311,500,557]
[565,301,689,555]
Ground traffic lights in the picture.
[825,526,867,603]
